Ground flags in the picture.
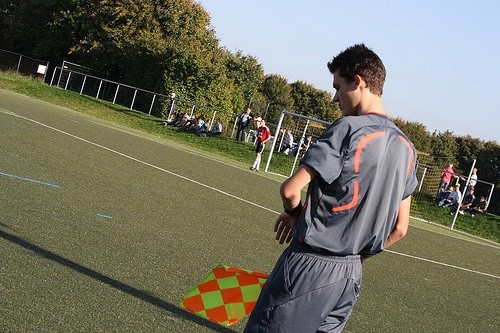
[177,263,269,328]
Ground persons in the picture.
[249,119,271,172]
[432,162,490,218]
[163,107,316,159]
[242,42,420,333]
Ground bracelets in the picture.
[283,201,304,216]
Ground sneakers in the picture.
[250,167,255,170]
[254,169,260,172]
